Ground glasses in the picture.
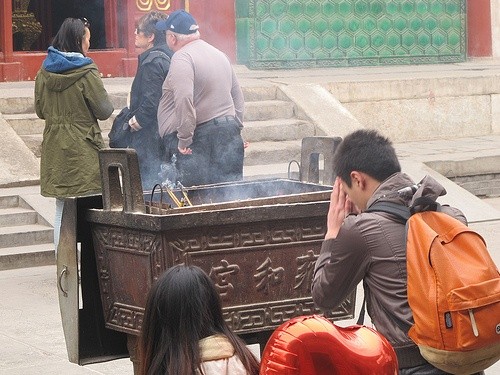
[137,27,144,34]
[80,17,91,26]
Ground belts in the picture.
[201,115,234,126]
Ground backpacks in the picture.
[364,196,500,375]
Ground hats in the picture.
[155,10,197,34]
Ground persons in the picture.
[34,17,114,283]
[155,9,248,187]
[311,129,487,375]
[108,10,179,191]
[136,263,261,375]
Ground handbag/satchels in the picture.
[108,107,134,149]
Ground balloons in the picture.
[259,314,399,375]
